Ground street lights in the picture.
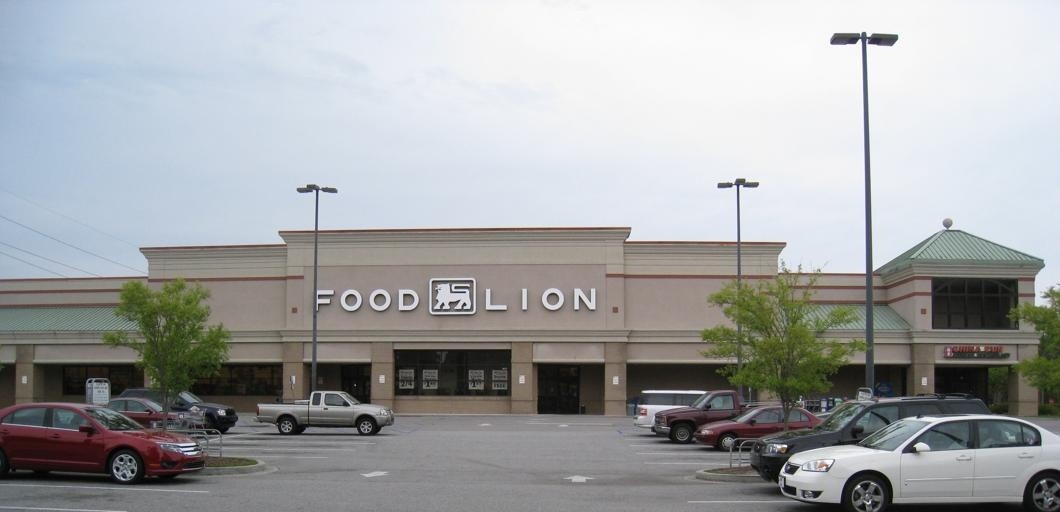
[295,181,339,398]
[827,28,902,399]
[718,178,763,407]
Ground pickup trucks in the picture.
[255,387,398,438]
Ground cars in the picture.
[0,376,239,483]
